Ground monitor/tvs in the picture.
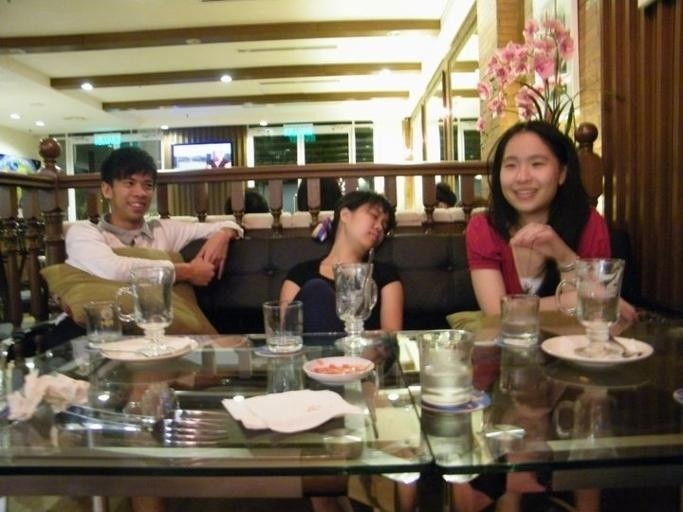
[171,138,235,172]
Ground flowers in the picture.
[477,15,579,137]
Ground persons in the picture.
[473,318,632,464]
[223,191,268,214]
[263,188,424,512]
[92,332,255,448]
[434,183,457,209]
[282,332,402,452]
[295,176,342,211]
[455,121,640,511]
[58,143,247,511]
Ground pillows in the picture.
[38,248,220,334]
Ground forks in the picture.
[56,406,232,447]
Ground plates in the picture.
[545,361,657,390]
[100,337,199,364]
[301,355,375,388]
[539,334,655,374]
[103,360,200,384]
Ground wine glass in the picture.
[120,380,182,447]
[332,264,379,352]
[114,260,177,357]
[547,390,613,465]
[554,256,626,360]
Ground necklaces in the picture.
[519,249,534,290]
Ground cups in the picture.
[499,347,545,398]
[495,294,542,349]
[265,354,305,395]
[81,301,122,350]
[0,341,10,419]
[262,299,305,355]
[417,405,476,471]
[416,330,475,409]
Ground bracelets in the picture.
[554,277,578,319]
[560,267,579,273]
[553,256,580,270]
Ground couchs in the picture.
[48,227,615,343]
